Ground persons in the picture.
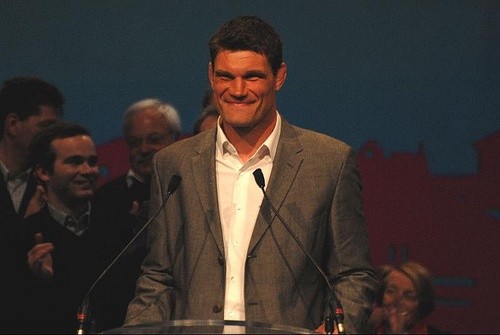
[94,98,184,331]
[194,106,219,134]
[363,261,441,335]
[95,16,374,335]
[0,122,142,335]
[0,72,65,222]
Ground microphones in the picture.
[77,175,183,335]
[253,168,346,335]
